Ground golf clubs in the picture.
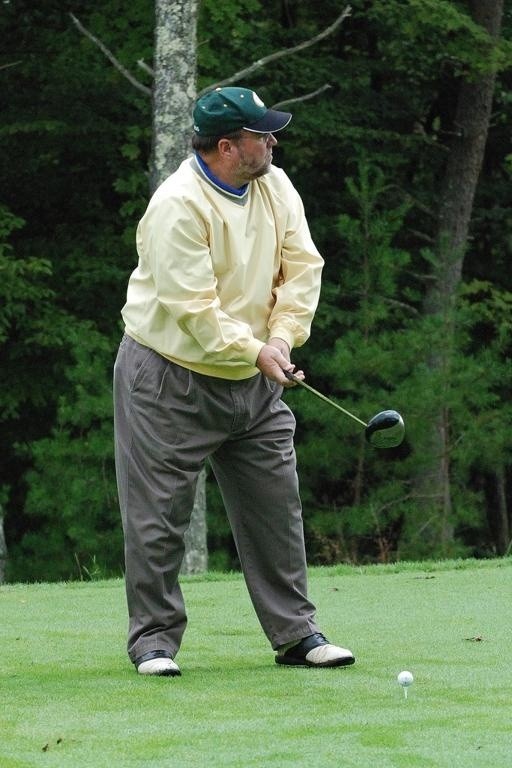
[282,368,406,449]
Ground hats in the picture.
[189,86,292,137]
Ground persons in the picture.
[113,85,355,676]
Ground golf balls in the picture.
[398,671,413,686]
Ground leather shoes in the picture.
[133,649,182,677]
[273,632,355,668]
